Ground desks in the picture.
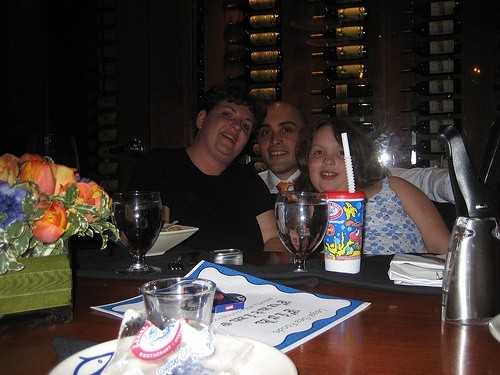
[0,251,500,375]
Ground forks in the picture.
[166,255,182,271]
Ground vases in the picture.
[0,254,73,317]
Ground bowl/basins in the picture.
[144,223,199,257]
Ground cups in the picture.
[319,191,365,275]
[140,277,216,332]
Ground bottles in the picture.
[440,217,500,326]
[196,0,462,173]
[85,0,118,176]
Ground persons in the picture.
[121,95,287,251]
[294,119,450,256]
[256,98,456,204]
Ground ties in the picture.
[275,182,294,202]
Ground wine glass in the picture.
[111,190,162,276]
[273,191,329,274]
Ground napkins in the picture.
[387,252,443,288]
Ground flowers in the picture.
[0,153,120,271]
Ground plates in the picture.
[488,314,500,342]
[49,333,298,375]
